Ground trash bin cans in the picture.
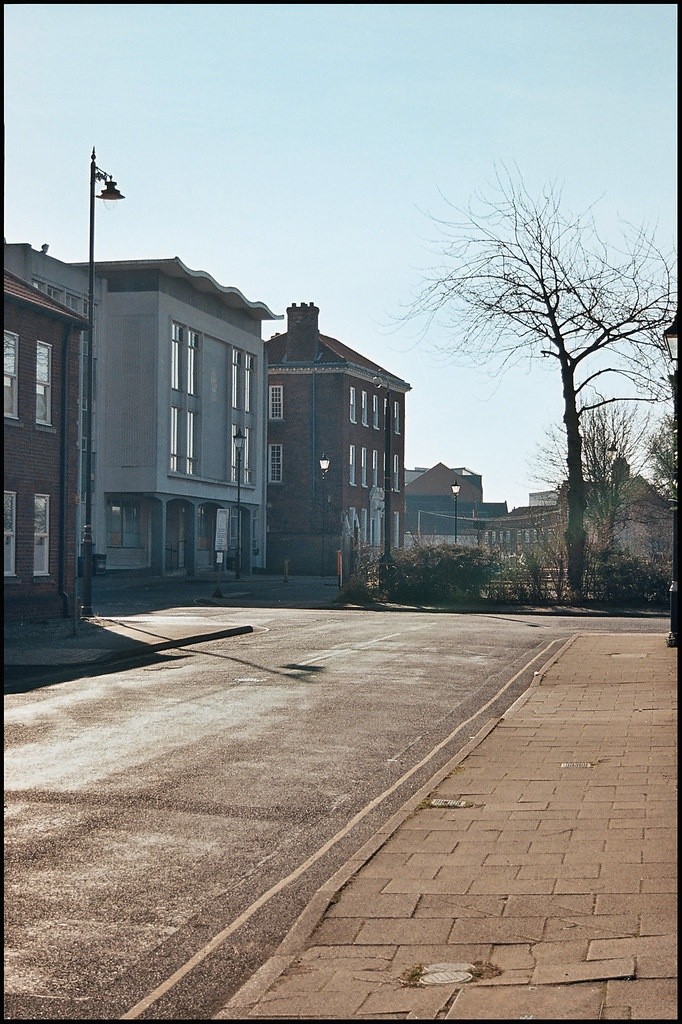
[93,554,107,576]
[78,557,84,576]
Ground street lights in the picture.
[232,427,246,577]
[373,368,397,591]
[319,453,330,577]
[452,481,460,542]
[608,440,622,555]
[663,313,681,647]
[79,166,127,615]
